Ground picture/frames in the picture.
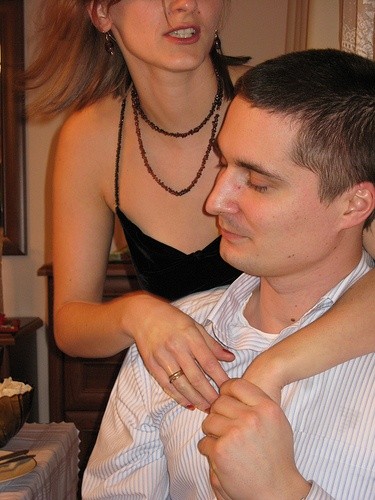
[0,0,27,257]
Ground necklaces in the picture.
[130,83,223,196]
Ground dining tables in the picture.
[0,421,82,500]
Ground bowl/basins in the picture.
[0,383,32,448]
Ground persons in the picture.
[7,0,374,415]
[79,48,375,500]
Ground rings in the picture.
[168,369,184,384]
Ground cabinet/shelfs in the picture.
[0,317,44,424]
[37,259,144,500]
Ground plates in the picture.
[0,451,37,483]
